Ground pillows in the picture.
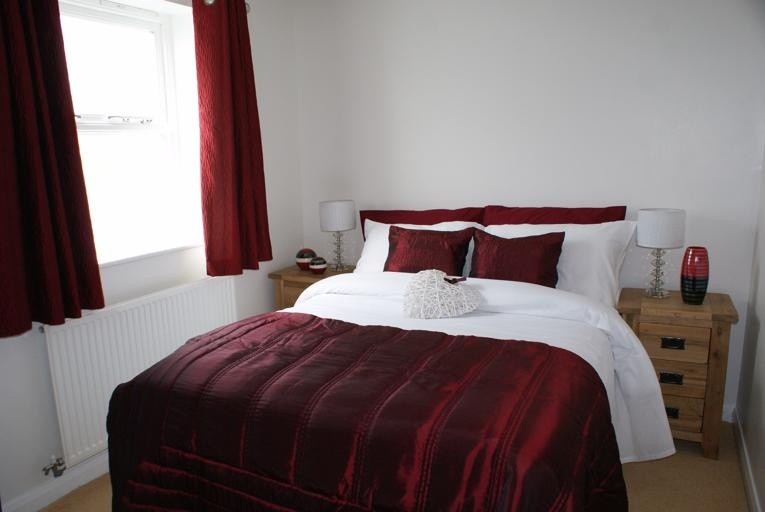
[354,206,637,320]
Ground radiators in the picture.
[38,275,238,477]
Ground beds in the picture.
[106,271,627,509]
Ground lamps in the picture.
[637,208,687,298]
[319,200,356,272]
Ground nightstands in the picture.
[617,286,739,460]
[267,264,356,311]
